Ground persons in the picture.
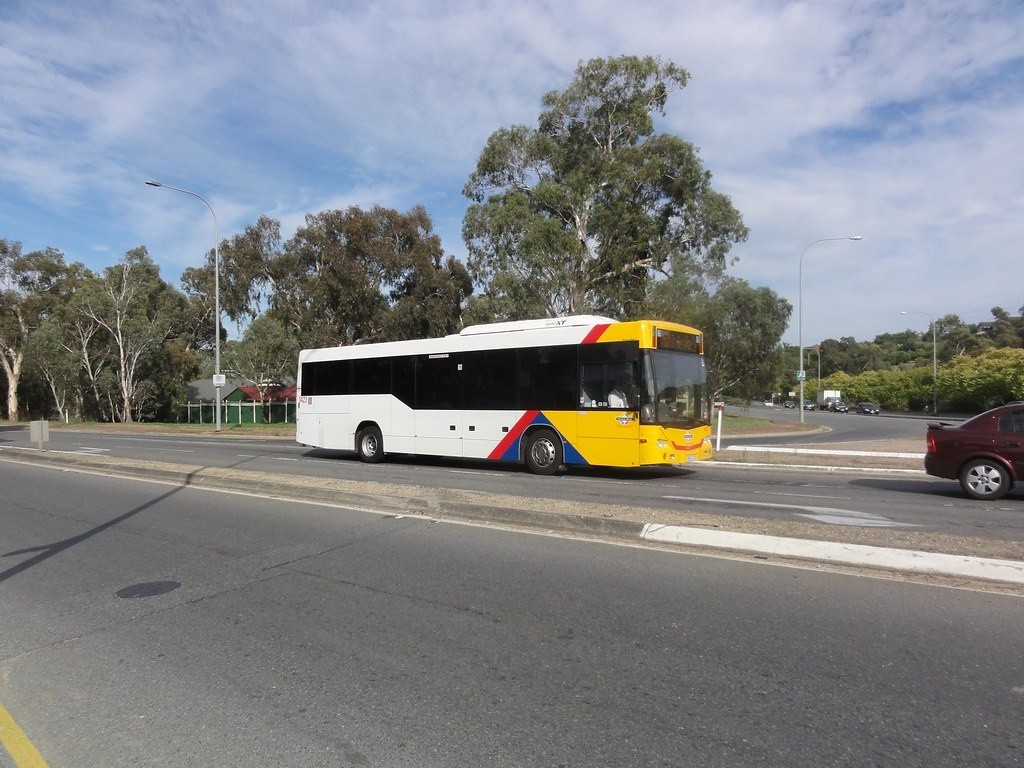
[608,372,631,408]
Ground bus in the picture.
[294,314,714,476]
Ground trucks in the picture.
[817,389,842,410]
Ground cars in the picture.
[856,402,879,416]
[924,403,1024,502]
[802,399,814,412]
[784,401,795,409]
[829,401,849,413]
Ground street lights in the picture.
[899,310,937,416]
[805,347,820,390]
[143,179,222,433]
[799,236,863,424]
